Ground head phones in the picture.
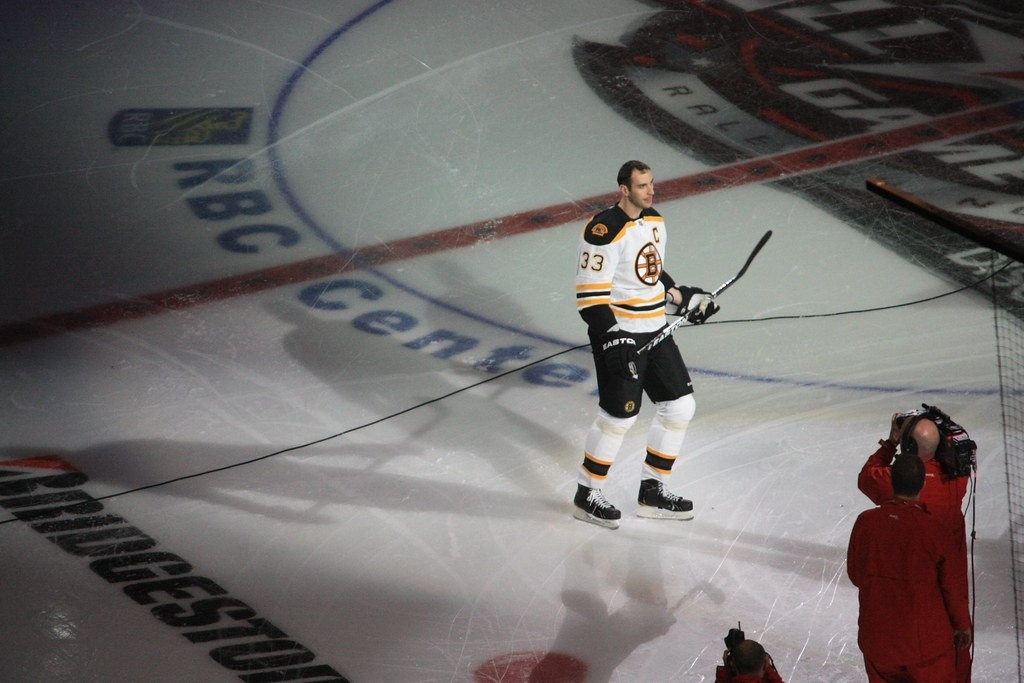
[900,413,934,456]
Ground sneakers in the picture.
[637,478,694,520]
[573,484,621,529]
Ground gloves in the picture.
[664,285,720,325]
[600,330,639,382]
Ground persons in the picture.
[715,639,786,683]
[847,453,973,683]
[857,412,971,683]
[525,530,673,683]
[572,160,720,529]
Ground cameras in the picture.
[723,628,745,663]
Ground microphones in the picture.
[921,403,952,424]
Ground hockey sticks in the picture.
[636,229,773,355]
